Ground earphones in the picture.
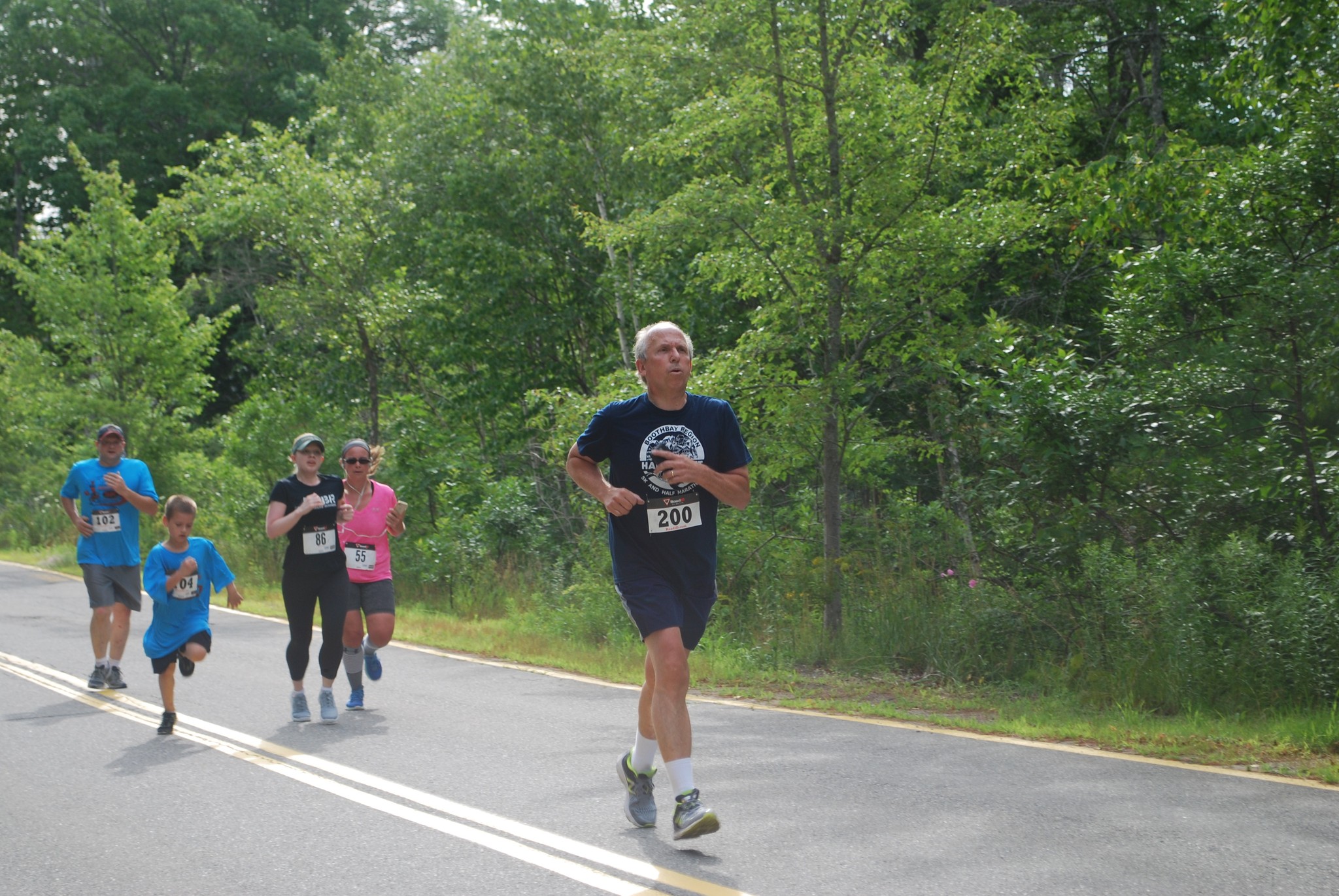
[369,463,372,467]
[342,463,344,470]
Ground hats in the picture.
[292,434,325,454]
[97,424,124,444]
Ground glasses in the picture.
[344,457,370,464]
[301,450,321,456]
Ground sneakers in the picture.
[346,689,364,710]
[363,640,382,680]
[672,788,720,841]
[157,711,177,734]
[291,693,311,722]
[617,749,657,828]
[318,691,339,722]
[106,666,128,689]
[178,655,194,676]
[87,665,108,692]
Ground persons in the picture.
[566,321,750,842]
[60,422,160,693]
[144,494,242,735]
[266,433,408,725]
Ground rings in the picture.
[671,470,674,477]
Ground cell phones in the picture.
[650,443,680,489]
[394,500,408,515]
